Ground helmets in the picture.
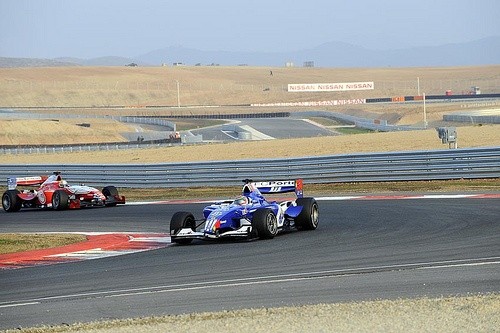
[235,195,255,207]
[60,180,71,188]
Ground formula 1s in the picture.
[169,177,319,245]
[3,171,126,212]
